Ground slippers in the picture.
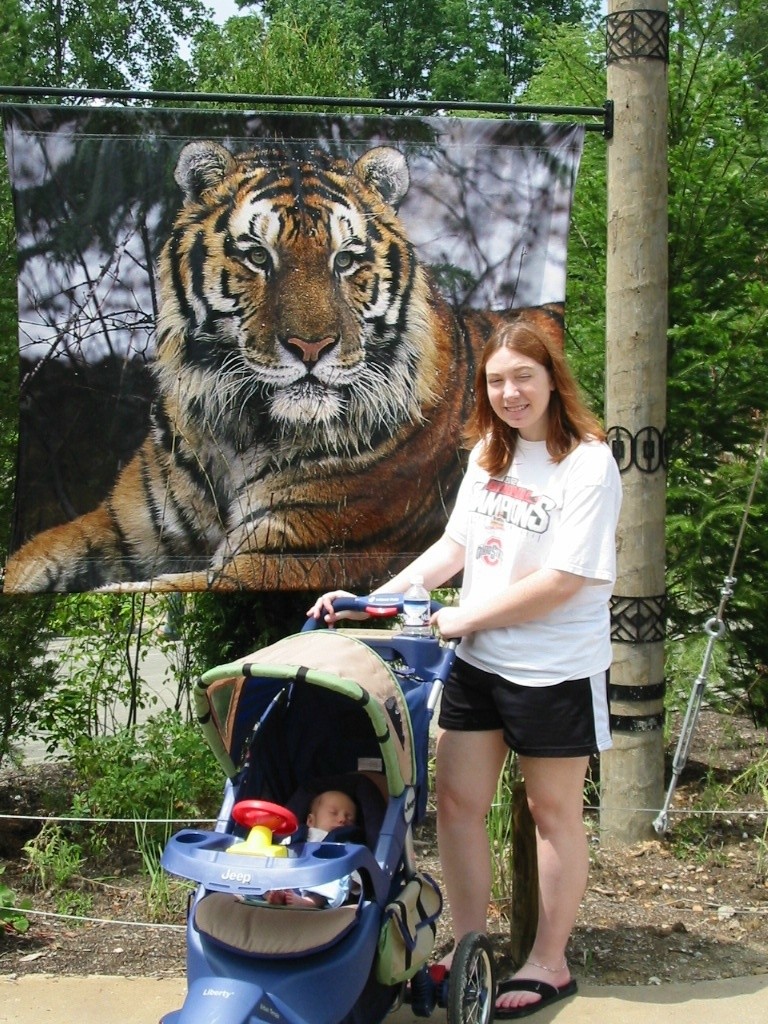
[492,979,579,1020]
[403,969,450,1004]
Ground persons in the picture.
[258,789,357,912]
[306,318,627,1022]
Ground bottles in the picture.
[401,574,432,642]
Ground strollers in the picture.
[160,589,497,1024]
